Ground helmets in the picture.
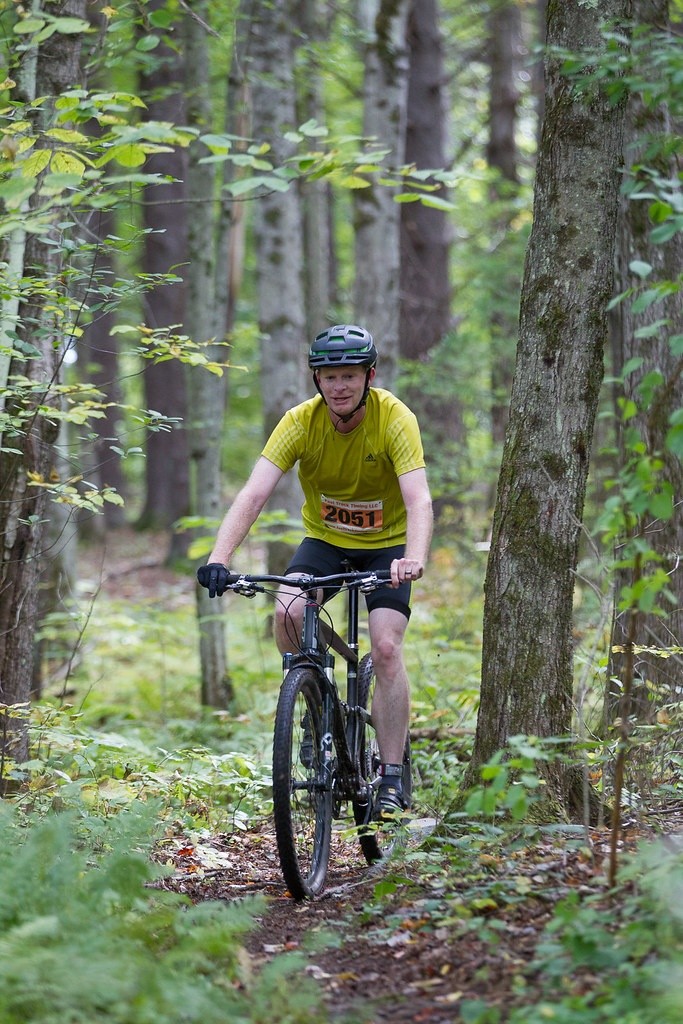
[307,323,380,369]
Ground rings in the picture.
[404,571,412,574]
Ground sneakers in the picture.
[367,788,405,834]
[299,709,315,770]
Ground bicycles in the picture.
[224,557,411,902]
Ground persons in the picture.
[197,321,434,828]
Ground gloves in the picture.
[195,562,231,598]
